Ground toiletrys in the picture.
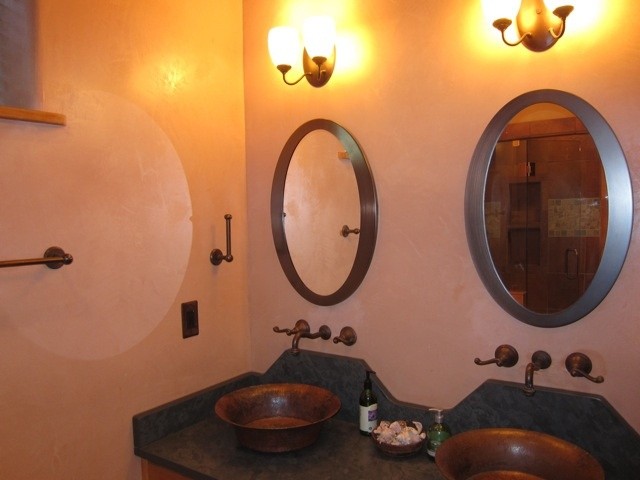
[427,408,452,458]
[359,370,378,436]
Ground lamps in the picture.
[485,0,600,53]
[268,15,362,86]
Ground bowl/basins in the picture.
[371,419,428,454]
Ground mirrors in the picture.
[269,118,378,307]
[463,89,635,329]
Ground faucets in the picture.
[291,325,332,355]
[522,350,551,397]
